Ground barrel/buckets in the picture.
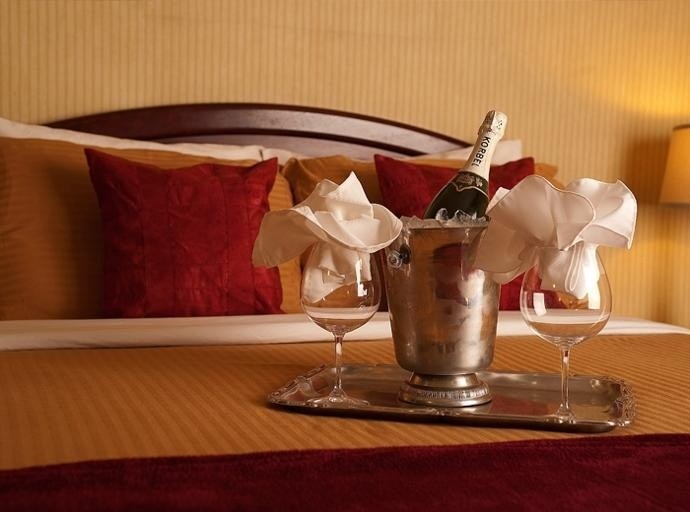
[379,224,501,408]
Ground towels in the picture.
[471,175,638,300]
[250,170,403,304]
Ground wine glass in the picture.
[519,246,613,426]
[300,242,383,407]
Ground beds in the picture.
[0,103,690,512]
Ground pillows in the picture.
[0,115,265,164]
[374,153,570,311]
[0,135,308,320]
[284,155,580,308]
[83,146,287,318]
[259,136,524,172]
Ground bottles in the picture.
[417,109,509,219]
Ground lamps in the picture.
[659,124,690,205]
